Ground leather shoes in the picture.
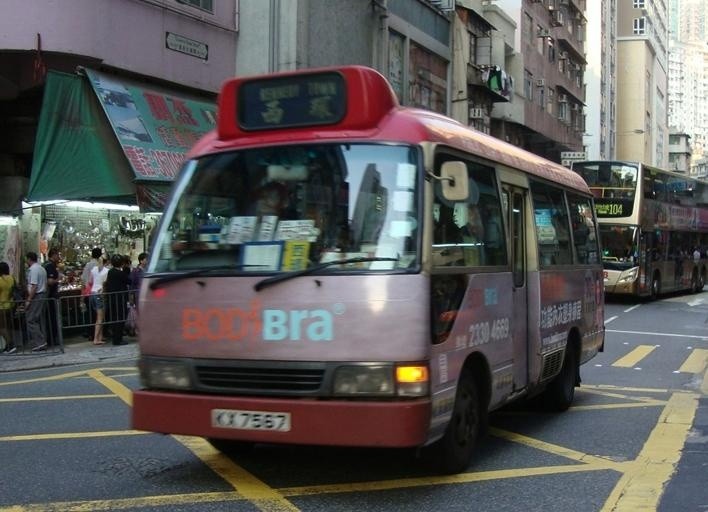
[3,347,17,354]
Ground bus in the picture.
[569,158,708,301]
[131,65,604,474]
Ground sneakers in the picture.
[31,342,48,351]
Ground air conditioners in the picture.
[535,79,544,87]
[550,10,564,27]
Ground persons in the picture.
[0,248,159,354]
[240,178,302,221]
[647,246,708,261]
